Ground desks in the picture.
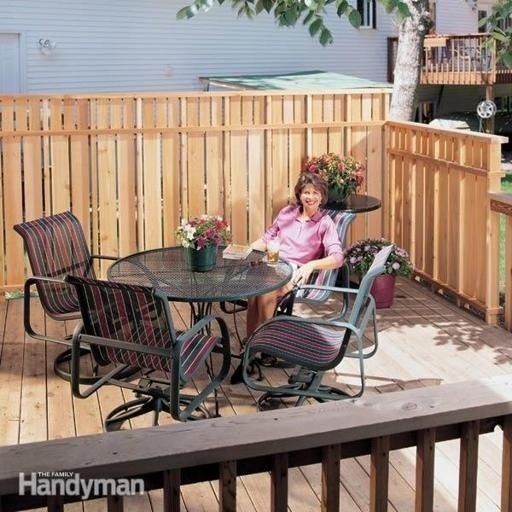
[320,193,382,290]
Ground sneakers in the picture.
[230,364,249,385]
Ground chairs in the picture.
[14,208,395,431]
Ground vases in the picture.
[371,274,394,309]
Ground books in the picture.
[222,243,252,260]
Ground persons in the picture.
[230,173,345,384]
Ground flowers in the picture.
[296,147,365,208]
[341,233,414,280]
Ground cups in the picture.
[267,237,279,266]
